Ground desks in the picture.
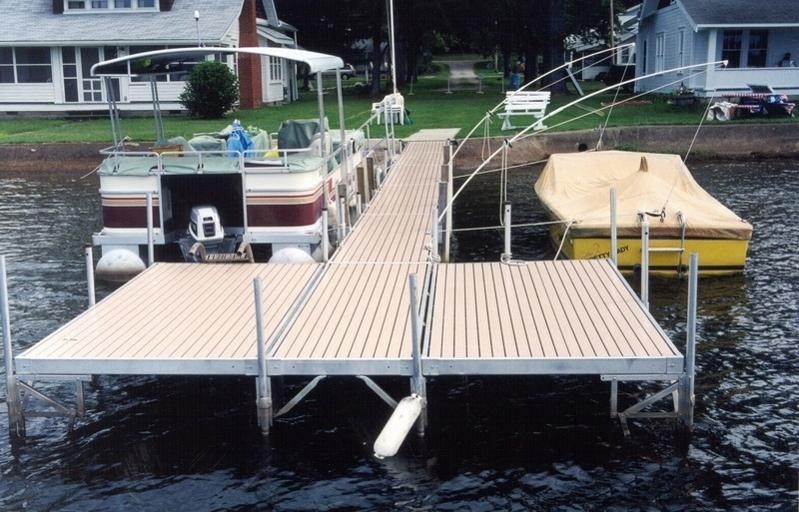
[721,92,785,120]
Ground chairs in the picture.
[371,90,406,126]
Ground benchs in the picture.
[751,101,795,117]
[497,89,553,129]
[732,103,761,119]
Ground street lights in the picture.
[194,10,204,47]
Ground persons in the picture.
[775,52,796,67]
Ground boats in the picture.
[532,148,754,282]
[87,45,383,274]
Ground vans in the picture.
[312,62,356,81]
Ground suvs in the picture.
[603,63,635,93]
